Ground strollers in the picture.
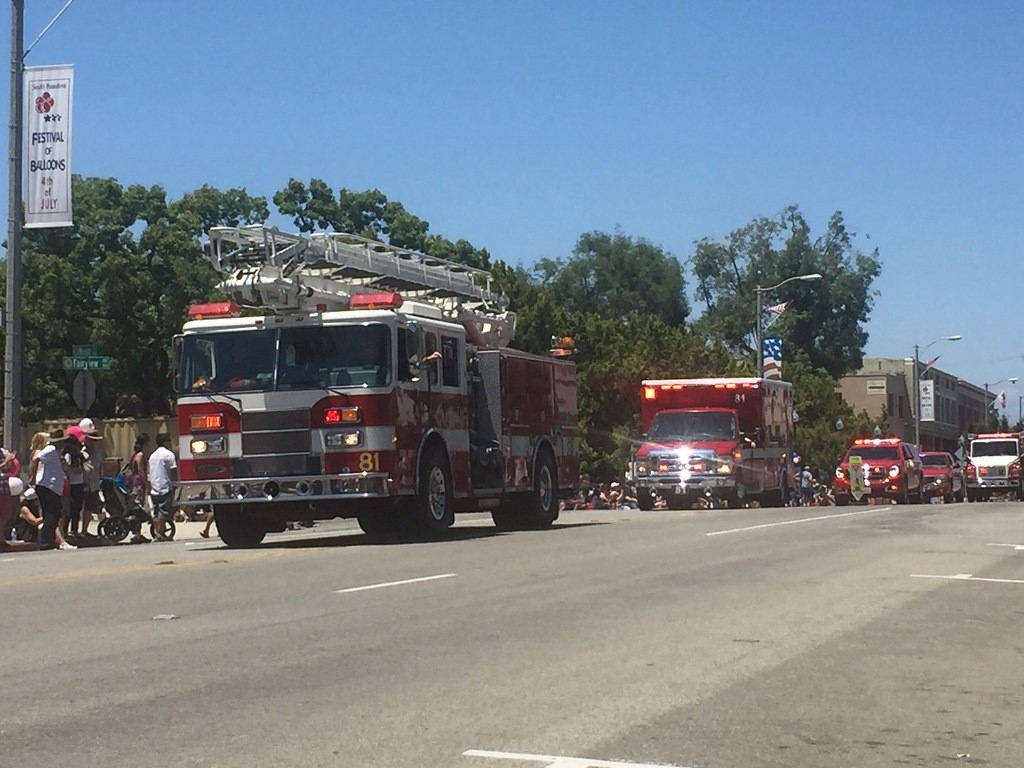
[96,472,176,542]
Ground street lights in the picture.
[913,335,963,450]
[753,273,823,380]
[984,377,1019,434]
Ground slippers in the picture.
[199,531,209,538]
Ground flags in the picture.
[926,355,939,366]
[762,336,782,380]
[998,392,1006,408]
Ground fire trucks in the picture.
[960,432,1024,503]
[165,223,583,549]
[631,375,793,510]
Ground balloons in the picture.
[792,452,802,464]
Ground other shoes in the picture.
[80,531,98,538]
[40,542,58,550]
[59,542,78,549]
[71,531,85,539]
[153,533,174,542]
[132,534,152,543]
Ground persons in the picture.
[692,495,728,509]
[558,481,667,511]
[789,466,835,506]
[0,418,216,551]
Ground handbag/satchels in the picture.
[120,462,133,479]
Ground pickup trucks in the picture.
[917,450,965,504]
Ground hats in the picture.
[85,430,104,439]
[611,482,619,487]
[67,426,86,444]
[23,488,38,500]
[48,428,69,442]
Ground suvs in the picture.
[831,437,926,507]
